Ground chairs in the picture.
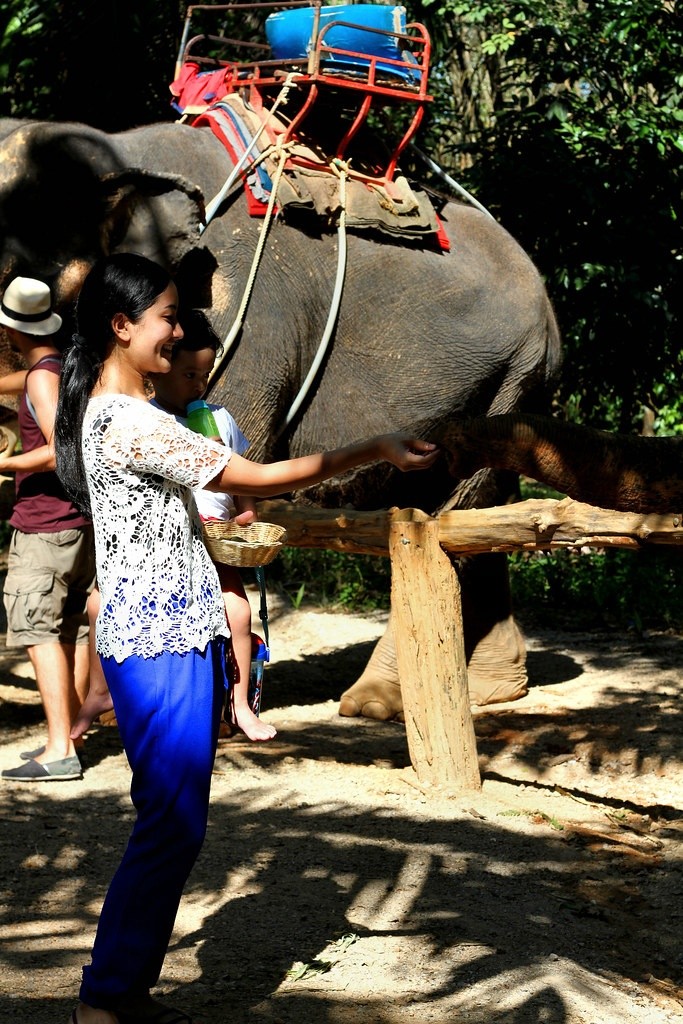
[170,4,428,194]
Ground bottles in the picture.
[186,400,223,443]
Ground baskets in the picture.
[204,520,287,567]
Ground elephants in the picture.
[0,111,564,724]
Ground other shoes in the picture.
[20,746,46,760]
[1,756,82,780]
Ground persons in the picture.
[67,309,278,741]
[56,253,439,1024]
[0,276,92,781]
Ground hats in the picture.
[0,276,63,337]
